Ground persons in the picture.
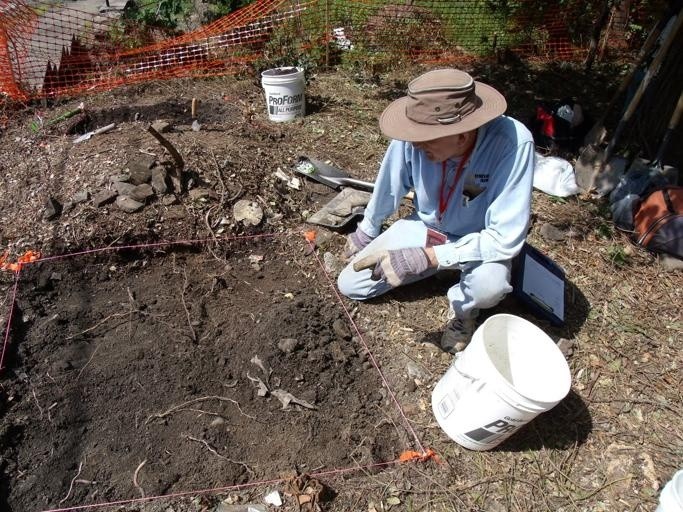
[339,66,536,354]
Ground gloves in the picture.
[353,247,432,288]
[343,227,375,258]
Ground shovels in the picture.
[289,154,415,199]
[305,186,396,228]
[563,3,682,208]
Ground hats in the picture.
[379,69,507,143]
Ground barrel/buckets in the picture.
[431,313,572,453]
[261,65,305,122]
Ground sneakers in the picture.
[441,318,478,355]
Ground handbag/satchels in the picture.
[632,186,683,257]
